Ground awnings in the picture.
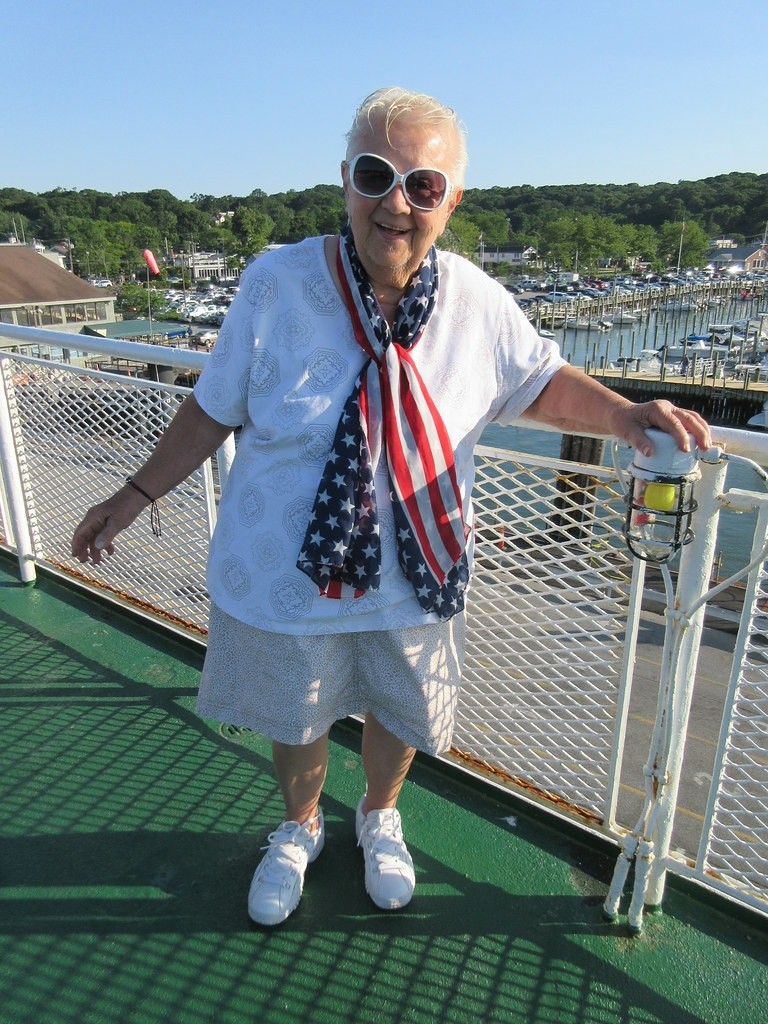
[84,319,187,338]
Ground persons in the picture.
[72,87,710,923]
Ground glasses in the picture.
[346,153,454,212]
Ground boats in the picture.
[611,318,768,381]
[592,312,635,324]
[562,316,613,332]
[647,293,765,312]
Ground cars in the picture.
[503,271,768,310]
[94,280,113,288]
[149,285,237,328]
[189,330,219,346]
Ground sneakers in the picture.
[356,790,416,909]
[248,804,325,925]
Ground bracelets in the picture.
[125,476,161,538]
